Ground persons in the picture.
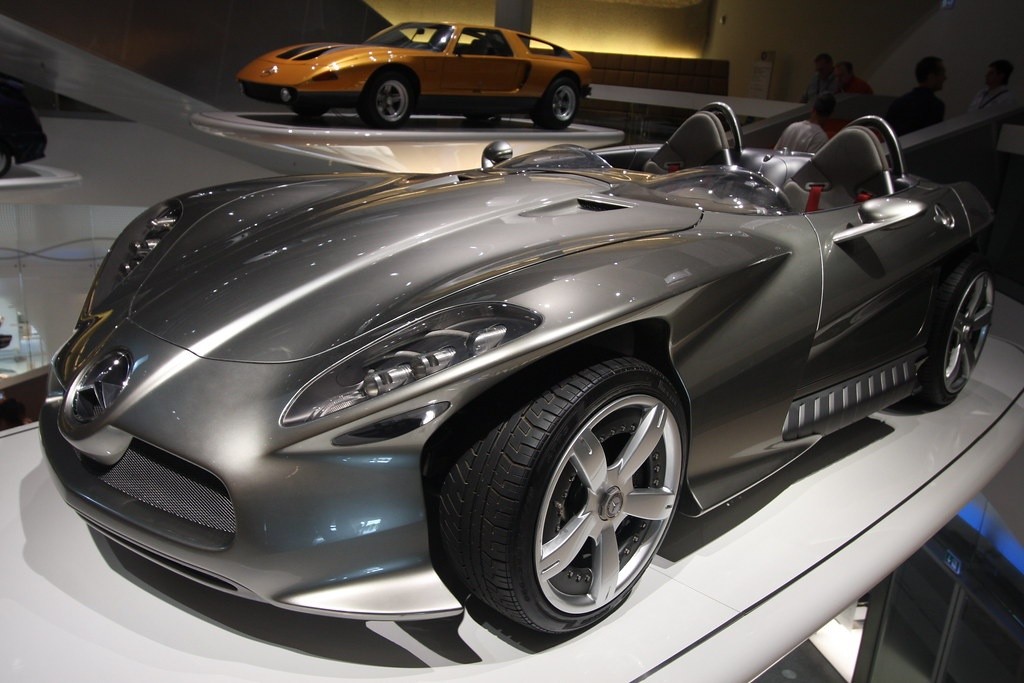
[882,56,948,185]
[798,53,839,104]
[774,92,836,153]
[979,59,1017,111]
[834,62,873,95]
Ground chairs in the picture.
[469,38,491,53]
[640,111,733,177]
[783,128,895,212]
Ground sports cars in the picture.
[35,98,992,637]
[234,18,594,131]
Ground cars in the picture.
[0,70,49,176]
